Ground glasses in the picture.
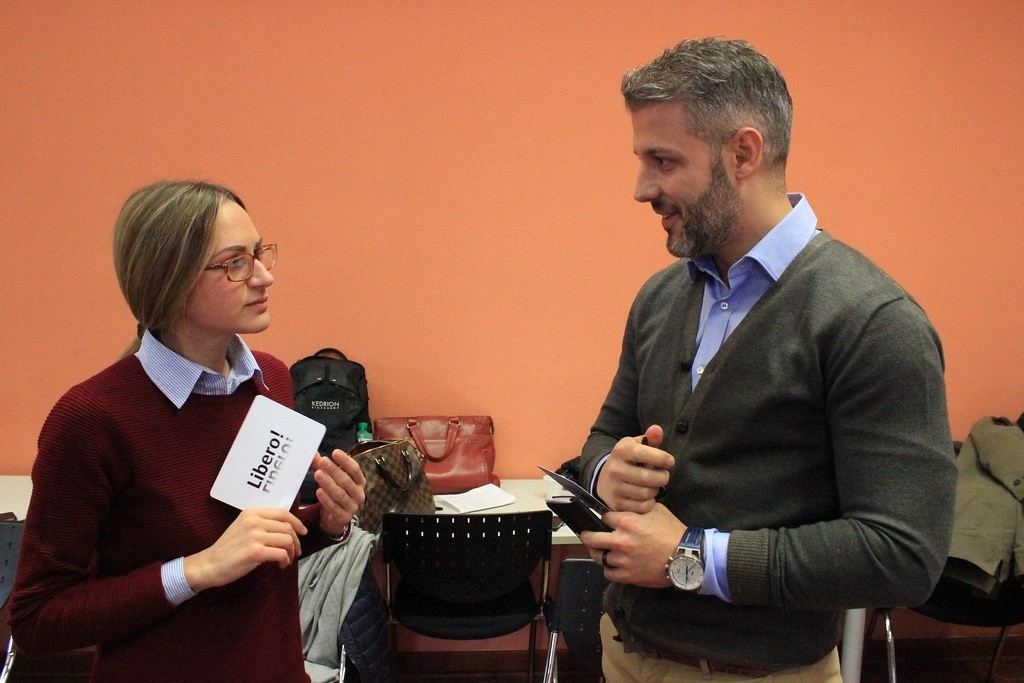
[203,244,278,282]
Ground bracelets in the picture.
[331,525,348,539]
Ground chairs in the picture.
[0,523,27,683]
[381,510,609,683]
[870,441,1024,683]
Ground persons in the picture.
[5,182,367,683]
[576,37,960,683]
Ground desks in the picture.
[0,475,601,544]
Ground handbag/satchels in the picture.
[336,438,435,534]
[374,414,500,495]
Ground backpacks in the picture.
[288,347,373,505]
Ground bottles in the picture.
[357,422,373,444]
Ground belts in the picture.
[612,632,773,678]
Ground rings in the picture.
[602,549,619,570]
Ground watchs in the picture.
[664,526,705,591]
